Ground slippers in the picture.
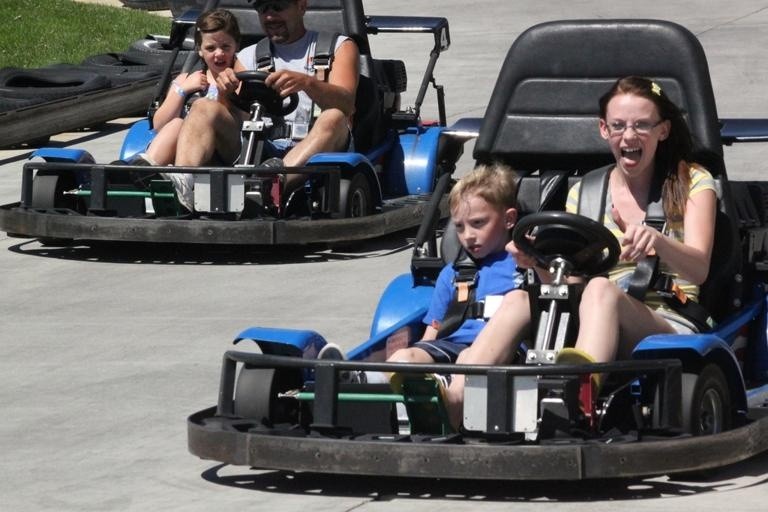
[387,369,458,436]
[553,348,604,413]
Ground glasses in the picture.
[605,117,664,136]
[253,1,294,15]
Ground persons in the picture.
[126,0,361,215]
[316,159,529,395]
[388,74,718,411]
[100,9,238,184]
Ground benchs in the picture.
[441,175,740,327]
[182,49,382,151]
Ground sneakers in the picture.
[255,157,287,203]
[316,342,367,385]
[106,153,196,215]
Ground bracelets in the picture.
[174,87,187,98]
